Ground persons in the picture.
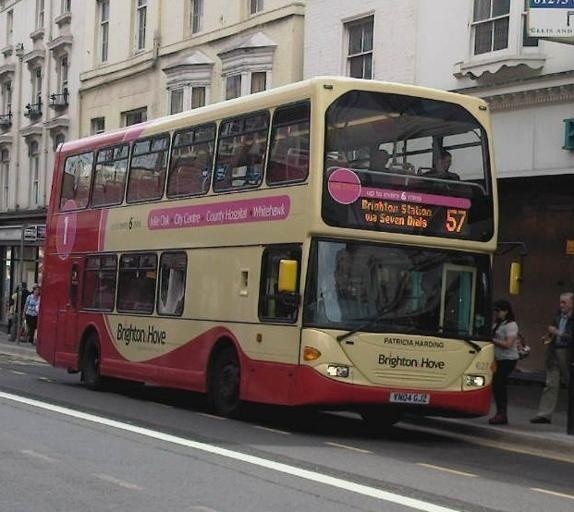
[370,149,391,172]
[408,273,452,332]
[7,282,42,343]
[489,298,519,424]
[427,149,459,179]
[529,293,574,424]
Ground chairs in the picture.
[390,162,433,176]
[63,148,310,208]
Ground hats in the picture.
[491,300,511,310]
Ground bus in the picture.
[35,75,527,425]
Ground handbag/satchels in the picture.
[516,337,531,359]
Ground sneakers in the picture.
[530,416,551,424]
[489,412,507,425]
